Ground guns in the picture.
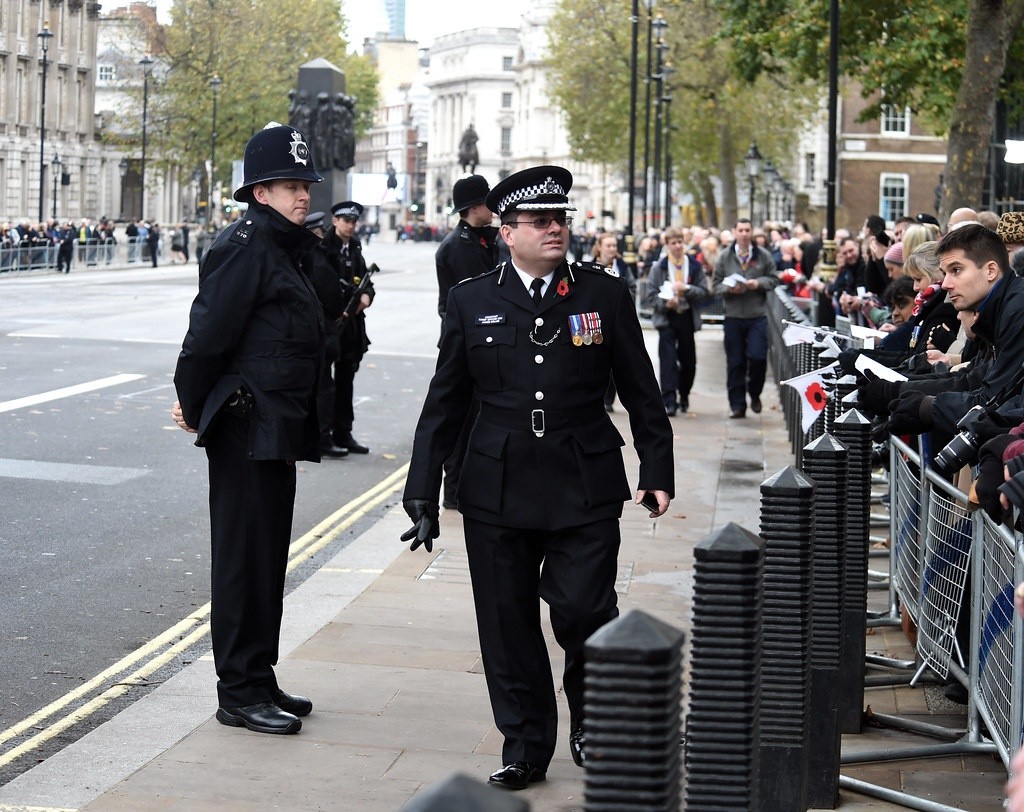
[323,263,380,355]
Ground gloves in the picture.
[401,499,440,553]
[885,390,936,437]
[856,369,905,419]
[838,348,877,375]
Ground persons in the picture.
[304,173,1022,812]
[172,122,326,735]
[0,216,223,274]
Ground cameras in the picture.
[934,404,1012,475]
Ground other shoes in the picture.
[681,401,687,412]
[322,437,348,458]
[945,682,967,706]
[335,433,369,454]
[730,411,746,418]
[668,406,676,416]
[607,404,613,412]
[752,397,761,413]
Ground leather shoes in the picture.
[216,701,302,734]
[271,690,313,717]
[487,759,547,790]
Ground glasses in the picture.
[506,216,574,229]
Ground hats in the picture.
[485,165,577,220]
[451,173,490,215]
[233,120,324,203]
[331,201,363,220]
[885,242,904,264]
[916,214,939,224]
[305,211,327,233]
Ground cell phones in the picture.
[641,492,659,515]
